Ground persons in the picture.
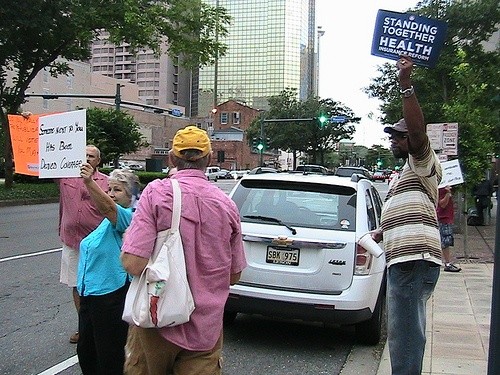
[22,110,110,344]
[371,55,444,374]
[438,185,464,272]
[76,163,142,375]
[119,125,247,375]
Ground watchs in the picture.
[399,85,414,98]
[446,192,452,197]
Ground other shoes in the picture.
[70,331,79,343]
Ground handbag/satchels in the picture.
[122,177,194,328]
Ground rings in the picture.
[401,60,407,65]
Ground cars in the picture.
[227,173,387,345]
[205,166,399,185]
[106,162,143,171]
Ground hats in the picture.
[384,117,408,133]
[172,125,211,161]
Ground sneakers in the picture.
[444,263,462,272]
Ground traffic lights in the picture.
[252,137,256,150]
[258,137,263,150]
[266,138,270,149]
[211,108,218,114]
[313,116,326,122]
